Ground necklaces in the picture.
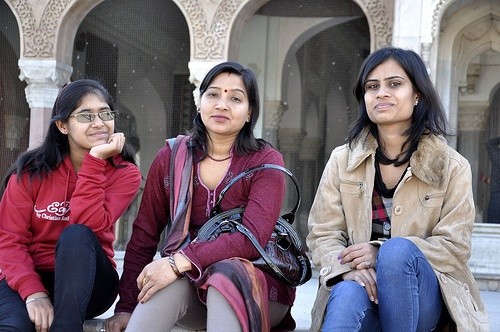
[207,154,231,162]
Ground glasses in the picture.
[68,111,116,124]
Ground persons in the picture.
[306,47,489,332]
[0,79,145,332]
[101,62,297,332]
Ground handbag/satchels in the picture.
[191,164,312,286]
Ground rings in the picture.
[143,278,147,286]
[366,261,371,269]
[101,328,106,332]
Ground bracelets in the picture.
[26,297,49,304]
[169,252,185,280]
